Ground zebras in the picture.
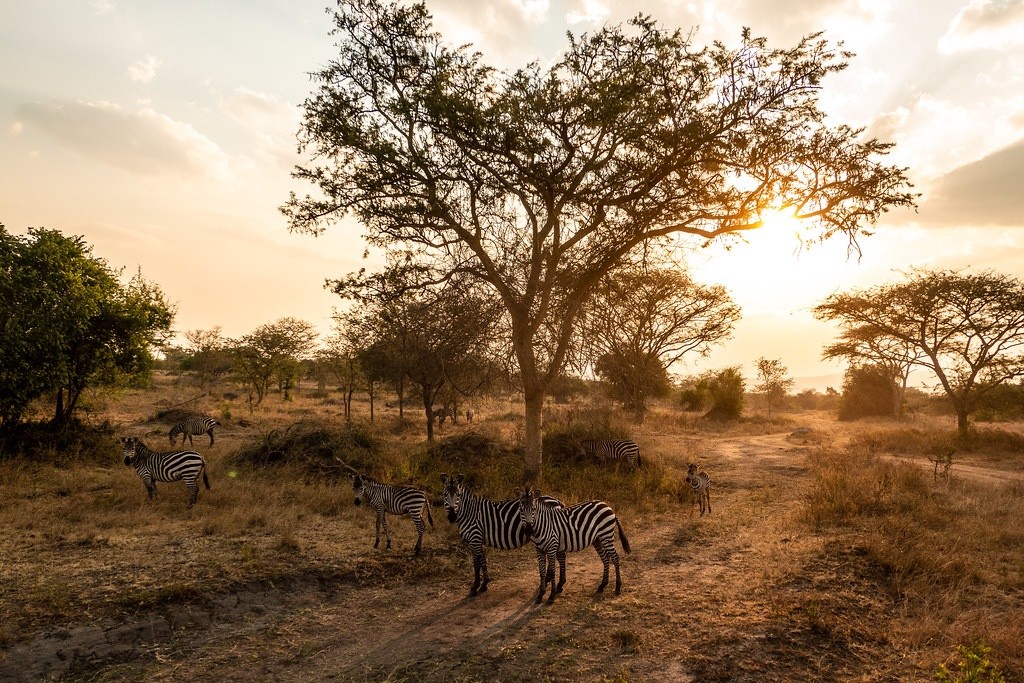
[442,475,630,604]
[121,436,210,509]
[169,415,215,449]
[576,438,642,474]
[686,463,711,517]
[351,473,434,553]
